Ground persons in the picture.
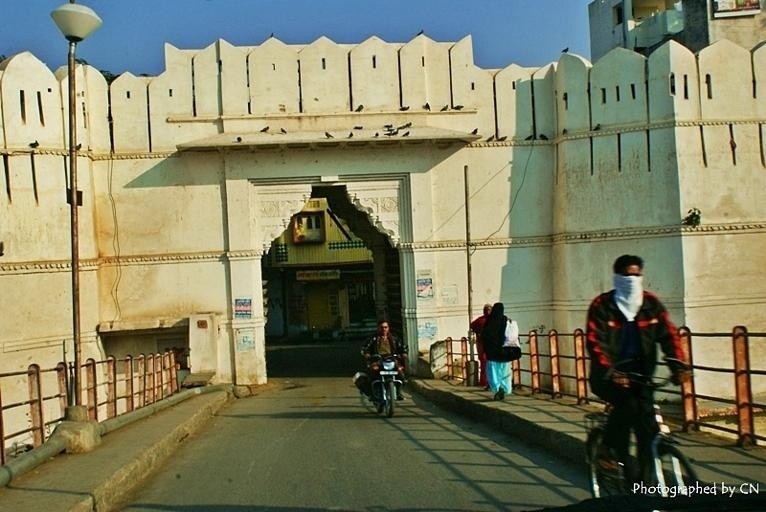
[480,303,513,400]
[586,255,690,474]
[470,305,493,390]
[333,315,345,342]
[363,319,409,400]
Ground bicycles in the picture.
[581,357,702,499]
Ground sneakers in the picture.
[594,444,626,481]
[482,385,507,402]
[367,393,406,402]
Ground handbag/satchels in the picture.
[503,319,521,349]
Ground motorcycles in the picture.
[359,343,409,413]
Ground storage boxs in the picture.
[351,372,370,394]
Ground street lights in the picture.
[50,4,102,462]
